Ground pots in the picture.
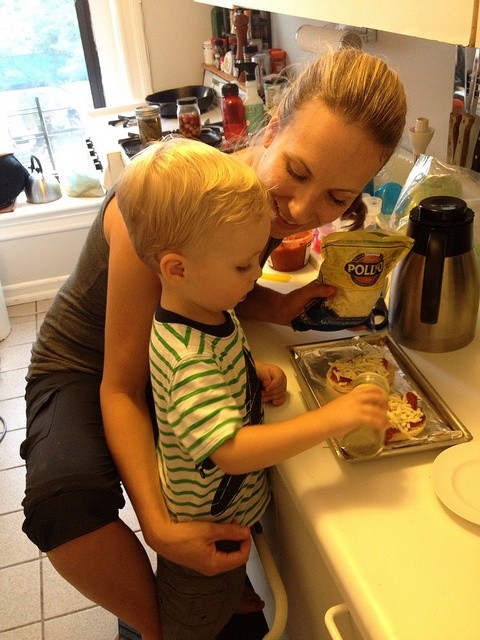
[145,86,216,118]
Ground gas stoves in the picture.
[89,71,249,162]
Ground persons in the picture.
[120,137,392,638]
[18,47,407,639]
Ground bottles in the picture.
[203,41,213,64]
[264,80,288,107]
[270,50,287,83]
[213,40,225,62]
[232,9,248,61]
[215,54,220,69]
[242,44,258,62]
[221,84,249,150]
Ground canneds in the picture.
[263,79,287,105]
[135,106,163,145]
[175,97,202,140]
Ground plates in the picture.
[430,442,479,528]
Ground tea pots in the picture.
[24,155,63,204]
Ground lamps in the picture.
[295,21,378,54]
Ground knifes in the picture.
[468,70,480,171]
[443,45,468,163]
[453,48,480,166]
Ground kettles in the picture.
[387,194,479,353]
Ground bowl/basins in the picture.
[266,230,315,273]
